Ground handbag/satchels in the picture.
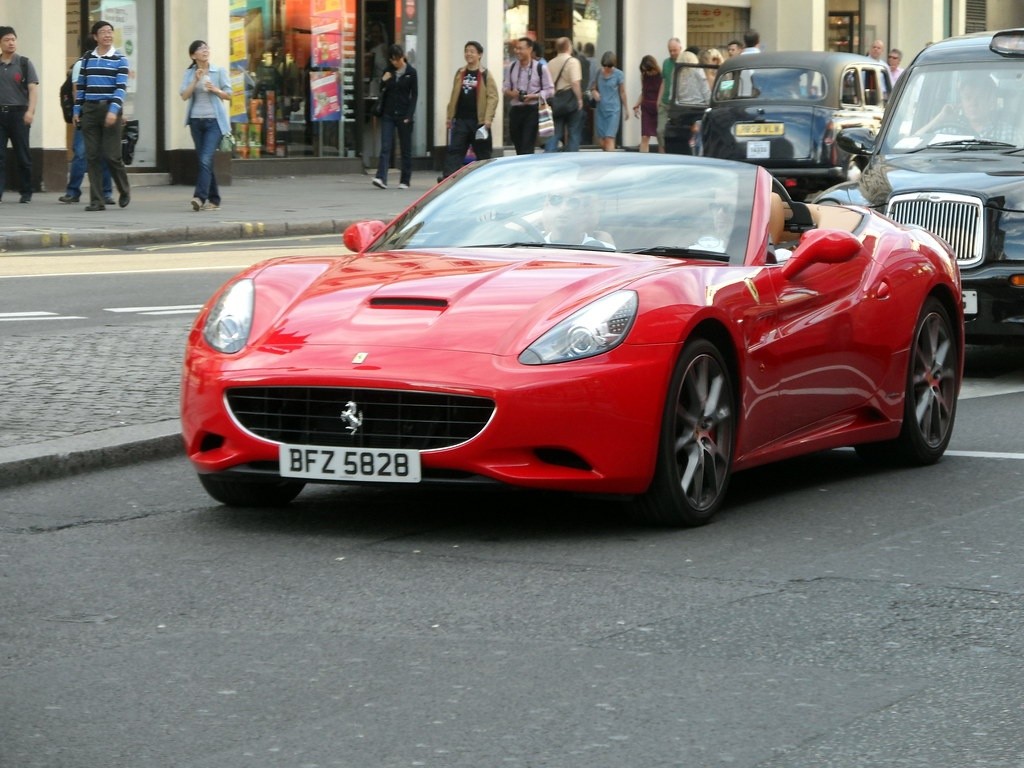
[537,92,555,138]
[372,67,392,117]
[589,68,602,108]
[547,87,576,117]
[121,119,139,165]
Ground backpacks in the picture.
[60,58,83,124]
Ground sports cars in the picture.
[181,151,965,529]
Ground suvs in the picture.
[803,28,1024,354]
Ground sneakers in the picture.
[120,194,130,207]
[372,177,387,189]
[85,202,105,211]
[191,197,202,211]
[59,194,79,202]
[397,182,408,188]
[20,195,30,203]
[204,202,220,211]
[105,197,116,204]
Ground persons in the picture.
[657,38,683,153]
[740,29,762,56]
[633,55,665,154]
[254,38,301,159]
[544,37,586,153]
[476,170,616,249]
[911,75,1024,148]
[684,175,775,265]
[179,40,233,211]
[571,43,598,142]
[668,46,712,155]
[59,21,130,211]
[591,51,629,152]
[867,39,890,91]
[887,49,905,90]
[700,48,725,92]
[437,42,499,182]
[0,27,39,203]
[727,41,743,59]
[372,45,418,190]
[502,37,555,155]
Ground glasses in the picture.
[708,203,734,214]
[194,46,209,51]
[550,193,580,208]
[888,56,898,59]
[603,64,614,68]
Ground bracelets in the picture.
[633,107,639,110]
[591,88,597,92]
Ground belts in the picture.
[0,105,28,113]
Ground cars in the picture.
[663,50,894,203]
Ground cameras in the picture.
[518,90,527,102]
